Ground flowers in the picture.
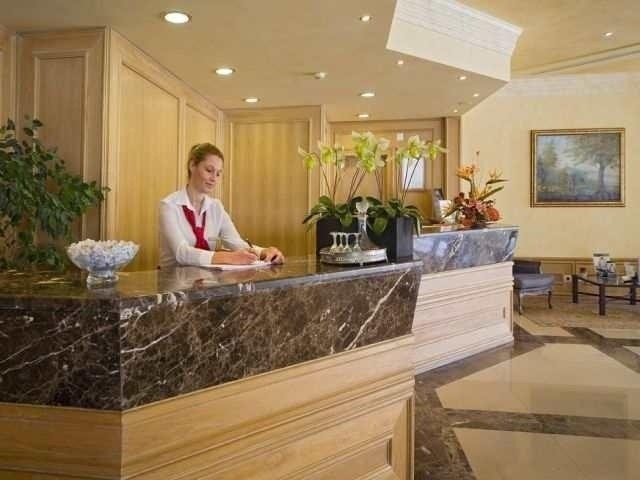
[440,149,509,224]
[351,129,445,239]
[453,193,487,226]
[296,127,388,230]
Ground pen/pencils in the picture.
[245,238,259,258]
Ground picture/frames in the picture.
[530,128,626,207]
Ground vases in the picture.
[366,215,415,260]
[461,219,486,229]
[315,216,363,260]
[484,204,501,223]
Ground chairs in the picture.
[510,260,553,317]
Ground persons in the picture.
[155,265,286,294]
[156,142,288,270]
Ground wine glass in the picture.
[328,231,363,253]
[64,242,141,286]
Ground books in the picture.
[197,261,274,272]
